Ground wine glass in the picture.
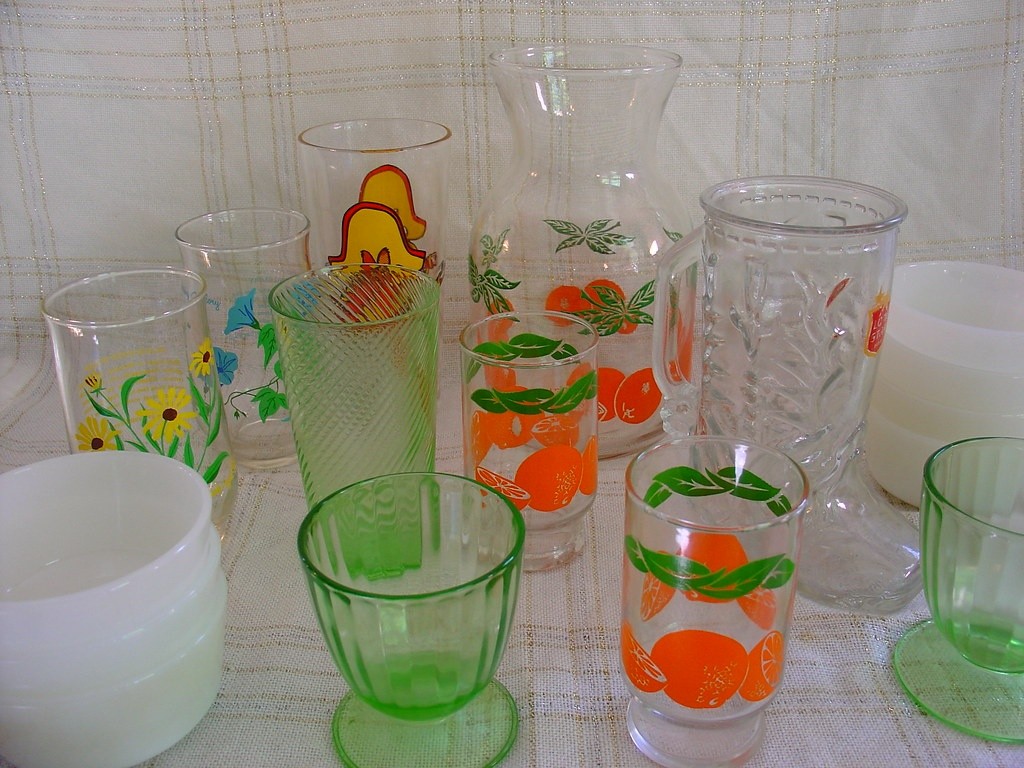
[892,437,1023,744]
[298,473,524,768]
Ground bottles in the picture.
[463,46,694,461]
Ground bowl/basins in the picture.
[1,451,226,768]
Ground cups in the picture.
[650,176,920,614]
[176,208,312,470]
[623,439,811,767]
[268,265,439,496]
[300,117,451,288]
[43,265,236,541]
[863,259,1024,509]
[458,312,598,574]
